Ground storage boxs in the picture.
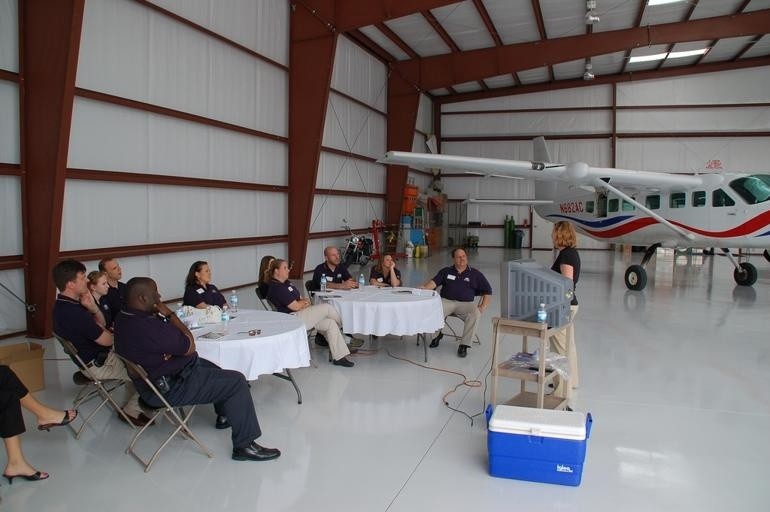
[0,341,48,394]
[485,402,594,487]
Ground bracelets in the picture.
[164,311,175,322]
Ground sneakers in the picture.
[346,338,364,347]
[348,348,358,353]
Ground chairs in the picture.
[305,279,316,305]
[254,286,278,312]
[438,294,484,346]
[113,351,218,473]
[50,331,137,441]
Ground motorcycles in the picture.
[338,215,376,271]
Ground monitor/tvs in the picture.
[499,258,574,327]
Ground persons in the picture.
[259,255,277,298]
[264,259,365,367]
[416,249,492,357]
[99,259,131,325]
[369,254,401,339]
[52,258,157,427]
[1,365,79,485]
[84,270,112,336]
[115,277,280,460]
[314,245,359,346]
[547,221,580,397]
[182,261,229,310]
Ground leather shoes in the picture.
[231,442,281,461]
[333,358,354,368]
[215,414,232,429]
[457,345,468,357]
[118,407,155,427]
[315,335,328,346]
[429,331,444,348]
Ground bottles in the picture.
[221,305,230,331]
[537,301,548,325]
[320,273,327,292]
[229,289,239,313]
[358,272,365,290]
[174,301,192,330]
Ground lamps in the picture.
[584,0,602,26]
[583,56,595,80]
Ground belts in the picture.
[80,363,93,371]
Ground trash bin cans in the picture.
[513,230,523,249]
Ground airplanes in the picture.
[377,134,770,293]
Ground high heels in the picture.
[2,471,49,485]
[38,409,78,433]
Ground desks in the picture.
[313,285,446,362]
[176,309,312,405]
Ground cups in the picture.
[352,271,359,289]
